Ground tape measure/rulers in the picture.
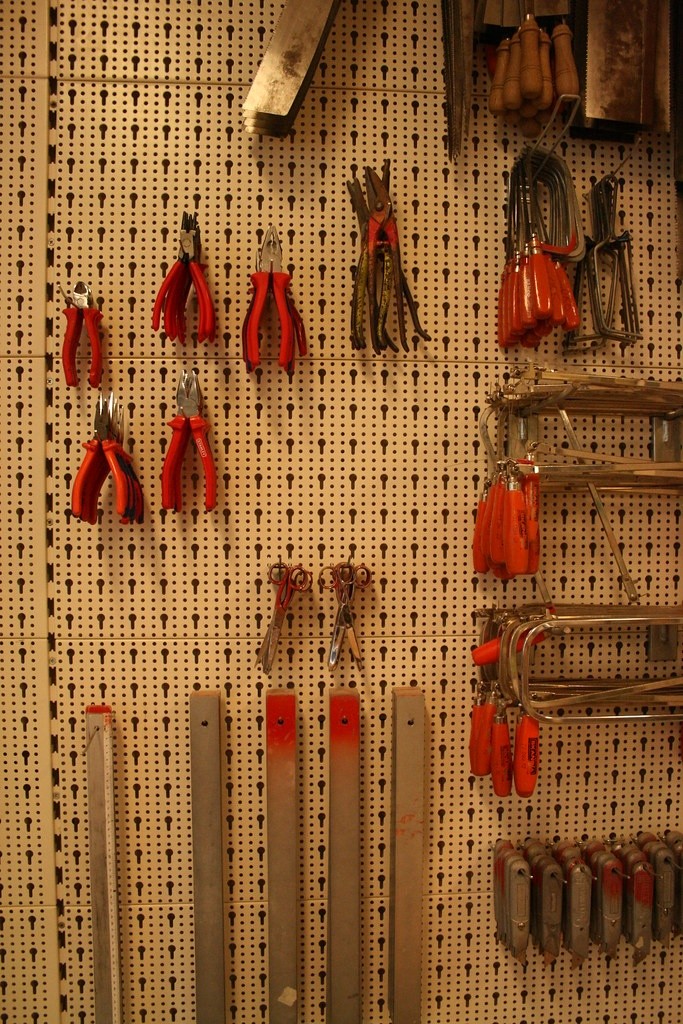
[85,706,124,1024]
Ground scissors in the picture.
[319,560,372,672]
[253,561,313,675]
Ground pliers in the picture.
[61,281,103,388]
[242,226,308,374]
[151,210,216,344]
[159,369,218,515]
[345,158,431,355]
[71,390,145,526]
